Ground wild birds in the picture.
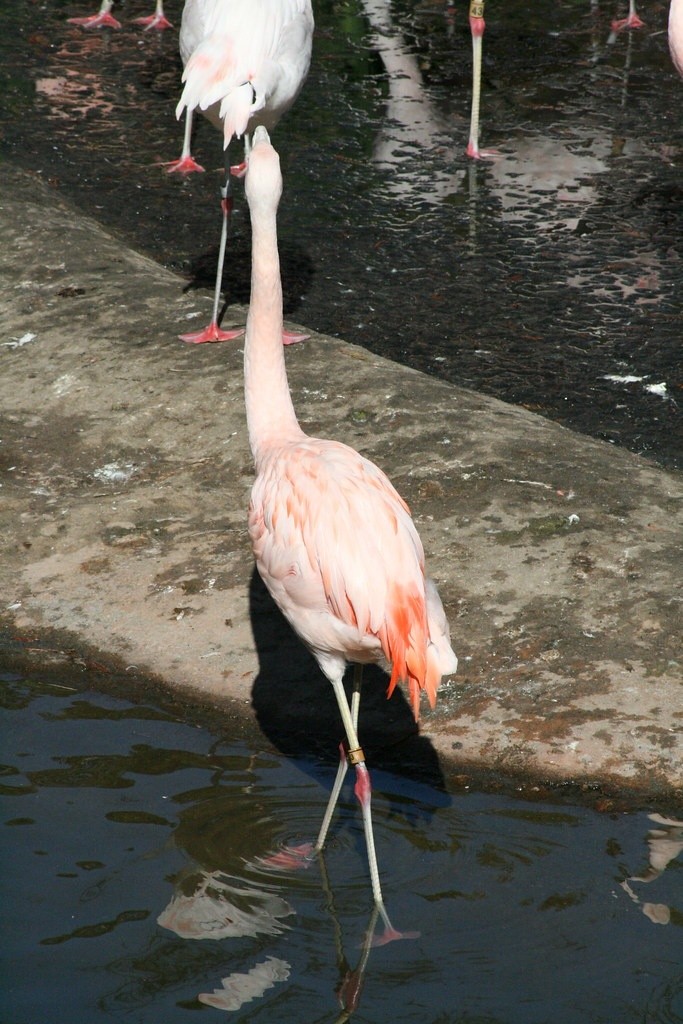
[67,0,683,348]
[241,123,460,950]
